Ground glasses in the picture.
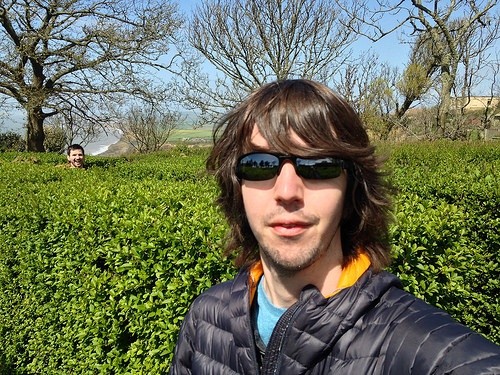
[234,151,348,181]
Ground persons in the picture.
[169,79,500,375]
[64,144,86,168]
